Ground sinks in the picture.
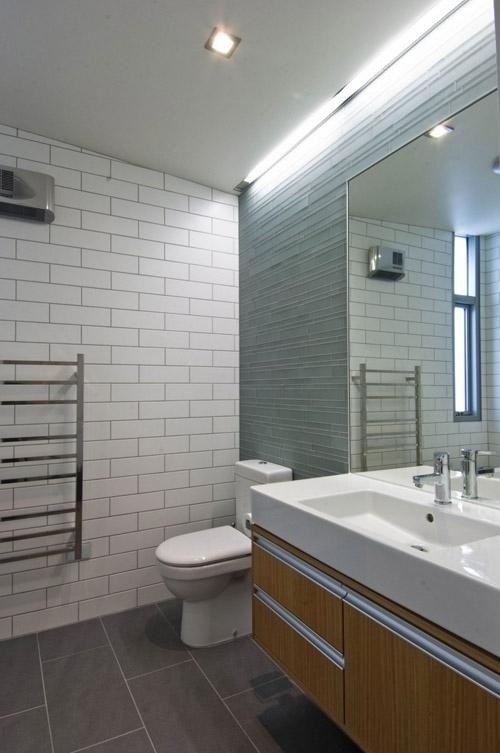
[301,490,500,548]
[452,475,500,503]
[242,513,251,538]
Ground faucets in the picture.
[460,449,500,499]
[413,451,451,505]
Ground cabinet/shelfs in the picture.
[251,525,499,752]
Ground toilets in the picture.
[155,460,293,648]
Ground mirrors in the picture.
[345,87,500,512]
